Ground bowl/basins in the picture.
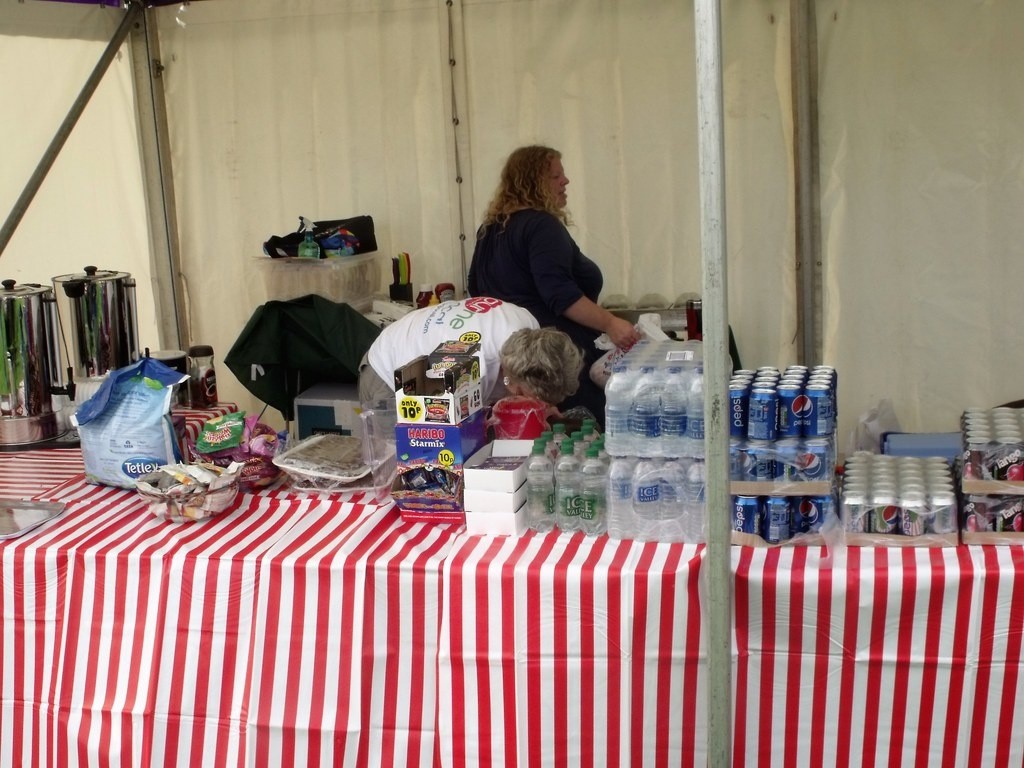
[137,469,240,524]
[216,460,283,490]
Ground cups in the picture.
[149,350,187,392]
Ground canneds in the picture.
[840,449,957,536]
[727,365,838,544]
[960,406,1024,532]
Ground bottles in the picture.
[188,344,218,410]
[525,418,607,536]
[416,283,433,309]
[605,338,707,543]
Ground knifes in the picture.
[392,251,411,285]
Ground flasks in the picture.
[685,299,702,341]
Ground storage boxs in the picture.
[467,502,528,535]
[393,341,483,425]
[463,482,528,513]
[464,438,535,492]
[255,251,381,313]
[392,406,486,524]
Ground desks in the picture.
[0,440,1024,768]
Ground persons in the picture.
[358,295,585,488]
[467,146,641,431]
